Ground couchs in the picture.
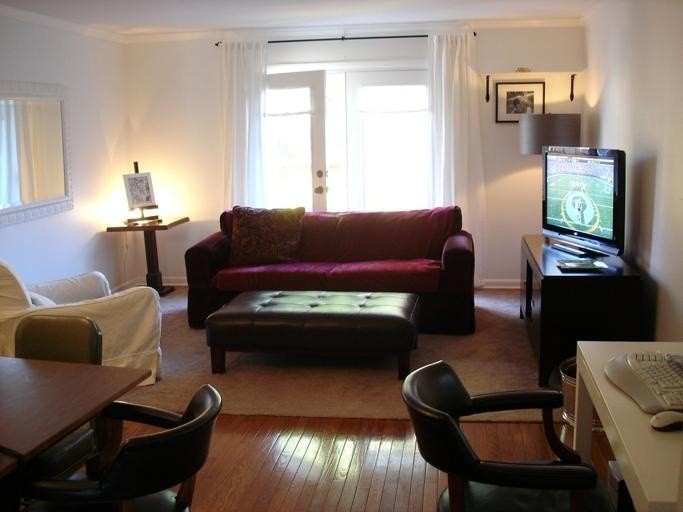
[0,260,164,387]
[203,289,420,378]
[184,207,477,337]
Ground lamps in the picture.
[518,112,581,154]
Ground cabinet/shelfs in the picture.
[518,233,656,389]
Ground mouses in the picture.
[650,410,683,431]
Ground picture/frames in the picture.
[121,172,156,210]
[495,81,545,124]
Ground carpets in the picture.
[114,285,566,422]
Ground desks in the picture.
[572,340,682,512]
[105,217,188,296]
[0,356,152,481]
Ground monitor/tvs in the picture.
[542,146,626,258]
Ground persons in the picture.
[547,159,613,220]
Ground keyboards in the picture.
[604,351,683,414]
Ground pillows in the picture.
[226,205,305,263]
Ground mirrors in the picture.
[0,80,76,233]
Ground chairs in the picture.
[401,358,597,512]
[15,314,102,366]
[22,382,222,511]
